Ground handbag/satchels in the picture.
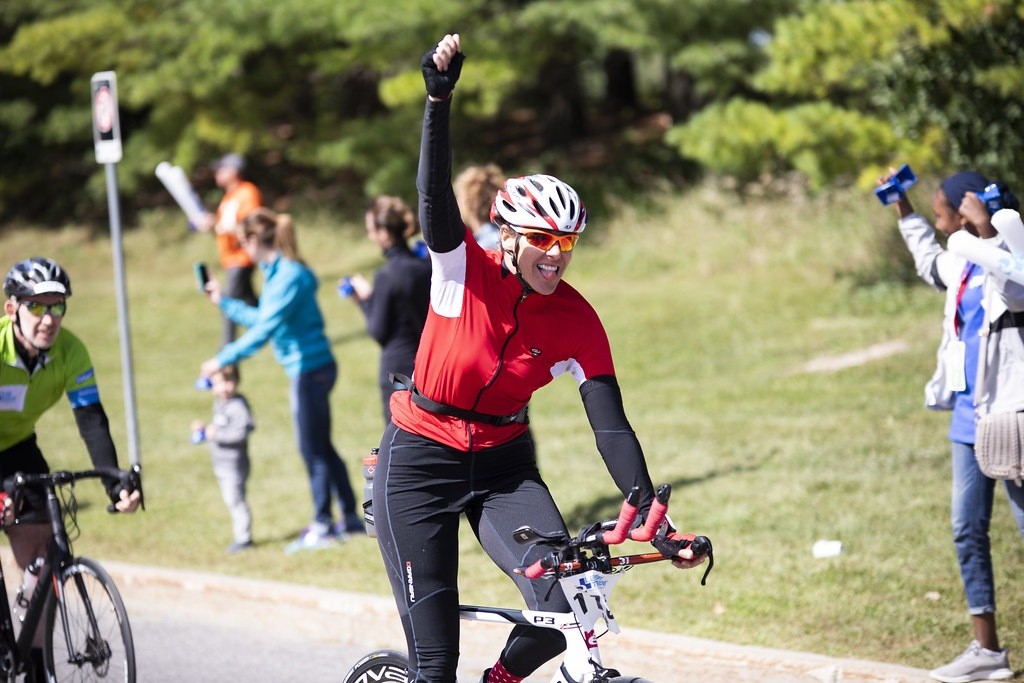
[975,412,1024,481]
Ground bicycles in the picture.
[342,483,714,683]
[0,461,147,683]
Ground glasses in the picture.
[17,299,66,317]
[509,225,579,252]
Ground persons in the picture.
[339,165,508,431]
[879,165,1024,683]
[194,151,265,390]
[373,33,711,683]
[0,256,143,683]
[199,209,366,553]
[191,362,256,554]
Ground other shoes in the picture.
[285,521,338,553]
[227,539,254,554]
[335,516,366,537]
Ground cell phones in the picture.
[198,264,209,285]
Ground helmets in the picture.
[5,257,72,299]
[490,174,587,233]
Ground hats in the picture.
[216,152,245,172]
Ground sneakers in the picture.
[925,640,1014,683]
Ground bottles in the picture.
[12,557,44,621]
